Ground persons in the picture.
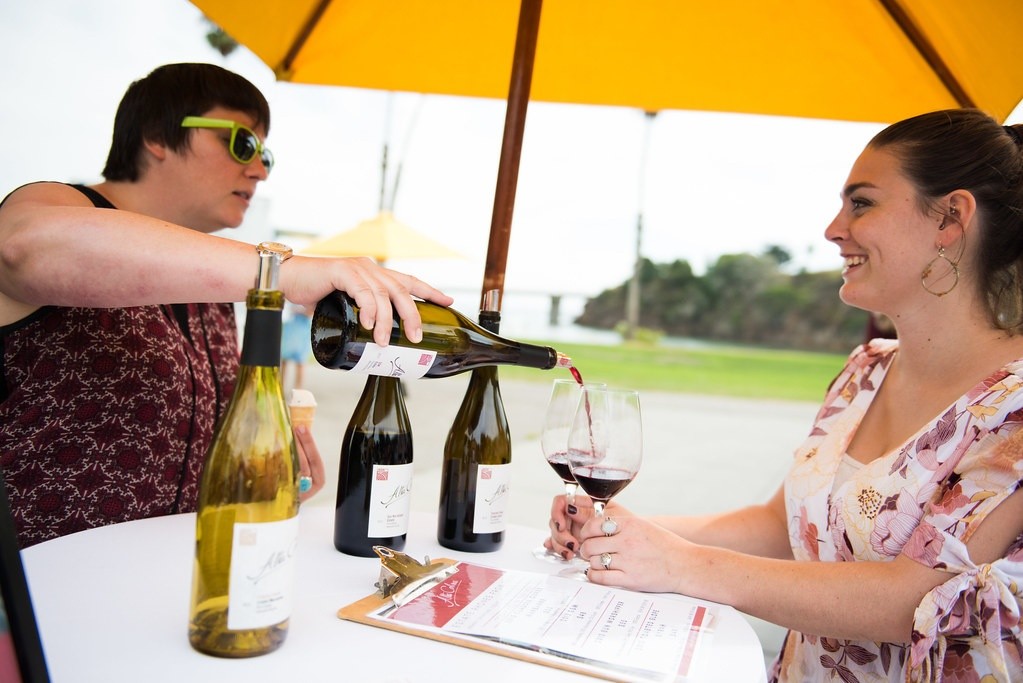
[543,110,1023,682]
[1,61,453,683]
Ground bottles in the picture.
[334,374,414,558]
[437,309,511,553]
[311,290,558,380]
[188,287,301,659]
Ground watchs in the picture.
[256,242,292,289]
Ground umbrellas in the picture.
[296,209,451,270]
[196,1,1023,313]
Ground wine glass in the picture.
[532,378,609,563]
[554,387,644,588]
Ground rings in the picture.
[601,553,612,572]
[599,518,619,537]
[299,475,314,493]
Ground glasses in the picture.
[180,116,274,175]
